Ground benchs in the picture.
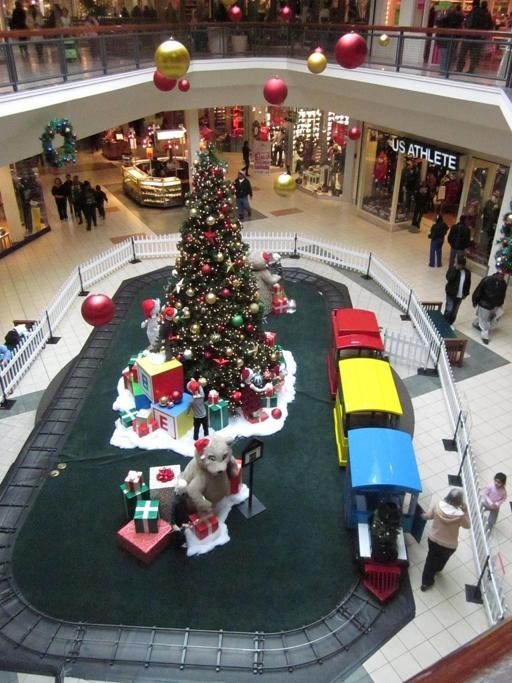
[421,301,468,368]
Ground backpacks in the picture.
[85,192,97,208]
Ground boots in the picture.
[238,214,244,219]
[246,207,252,217]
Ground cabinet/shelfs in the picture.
[122,164,181,208]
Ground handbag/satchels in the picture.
[492,307,503,328]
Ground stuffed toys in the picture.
[247,249,282,318]
[241,366,273,416]
[156,434,238,526]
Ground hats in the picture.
[239,171,245,177]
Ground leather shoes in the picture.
[472,322,481,331]
[483,339,489,345]
[421,582,433,591]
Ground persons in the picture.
[0,322,33,383]
[13,1,371,57]
[140,296,163,347]
[374,151,506,240]
[443,255,470,324]
[447,215,472,267]
[272,129,286,168]
[171,480,197,544]
[427,216,449,268]
[433,0,492,73]
[295,137,340,177]
[52,175,108,232]
[472,270,508,344]
[421,489,470,593]
[230,170,253,220]
[185,386,208,439]
[240,140,251,176]
[481,473,507,536]
[147,151,178,176]
